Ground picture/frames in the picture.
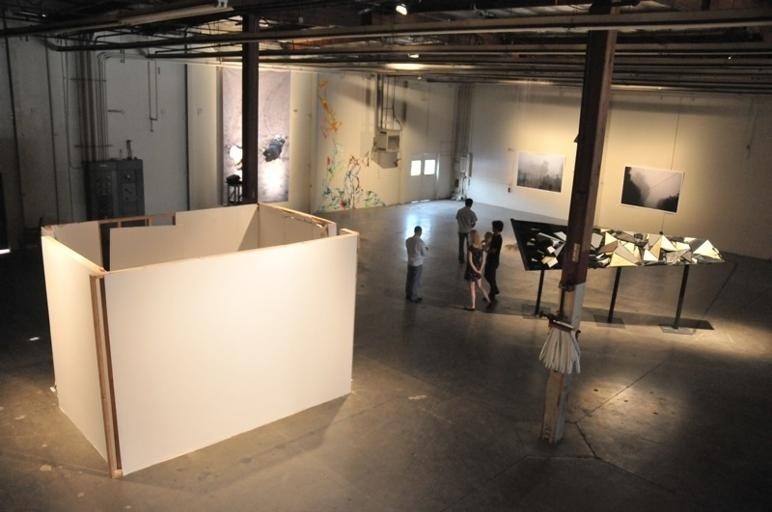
[515,151,567,195]
[619,164,685,214]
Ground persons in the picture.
[481,232,492,252]
[405,227,429,303]
[455,199,479,264]
[484,221,504,302]
[464,230,498,311]
